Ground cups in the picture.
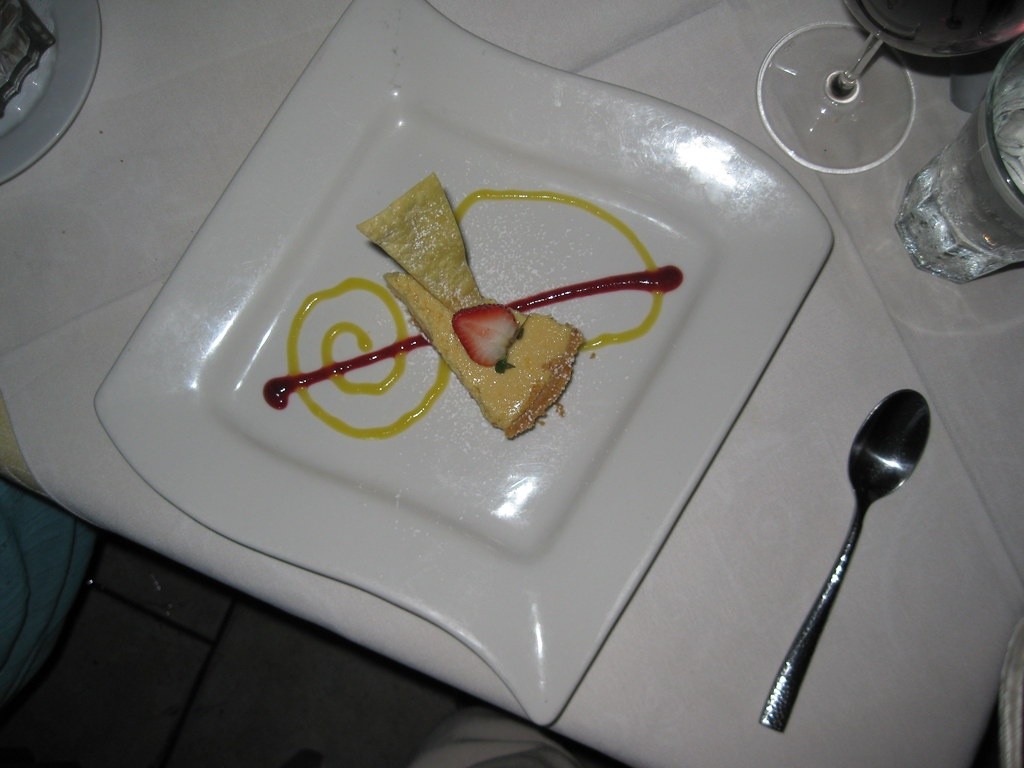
[894,35,1024,284]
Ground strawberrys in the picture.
[451,302,518,365]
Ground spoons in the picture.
[758,389,931,734]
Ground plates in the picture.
[0,1,104,188]
[91,0,840,732]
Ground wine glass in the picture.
[754,0,1024,178]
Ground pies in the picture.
[355,173,485,312]
[381,271,584,439]
